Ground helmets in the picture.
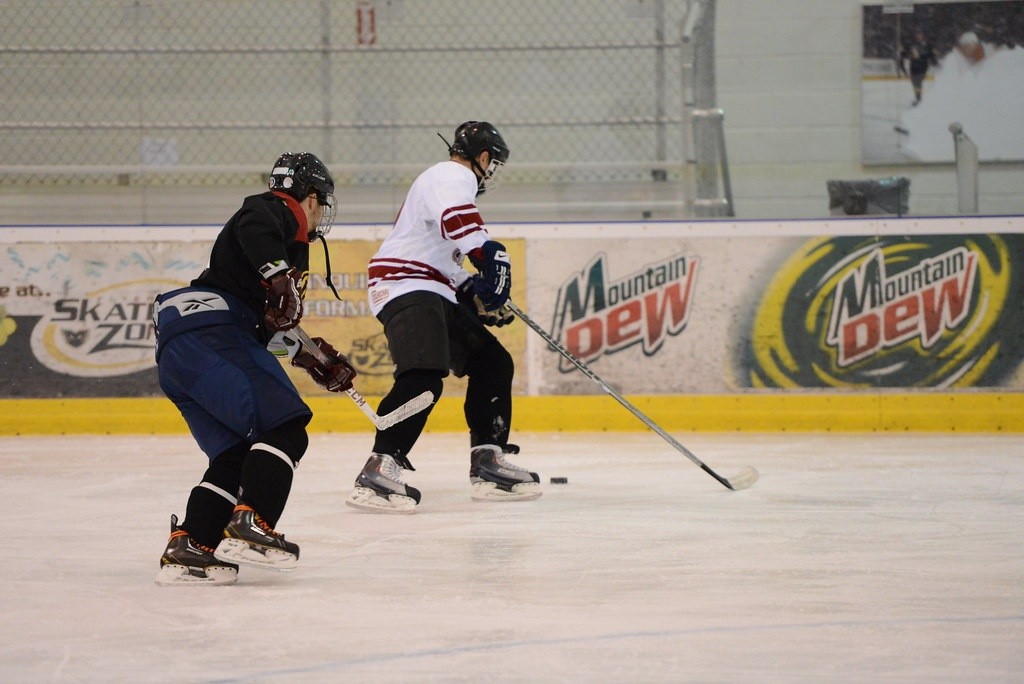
[447,120,510,197]
[267,152,334,243]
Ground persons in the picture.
[346,121,541,511]
[152,150,356,588]
[898,23,937,104]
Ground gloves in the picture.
[260,267,306,332]
[291,337,357,393]
[455,273,515,328]
[472,240,511,313]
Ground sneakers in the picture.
[469,443,543,502]
[345,451,421,513]
[155,513,239,584]
[213,485,300,572]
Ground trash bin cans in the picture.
[826,177,912,218]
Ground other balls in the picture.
[550,477,569,484]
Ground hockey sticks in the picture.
[292,325,435,432]
[503,299,761,492]
